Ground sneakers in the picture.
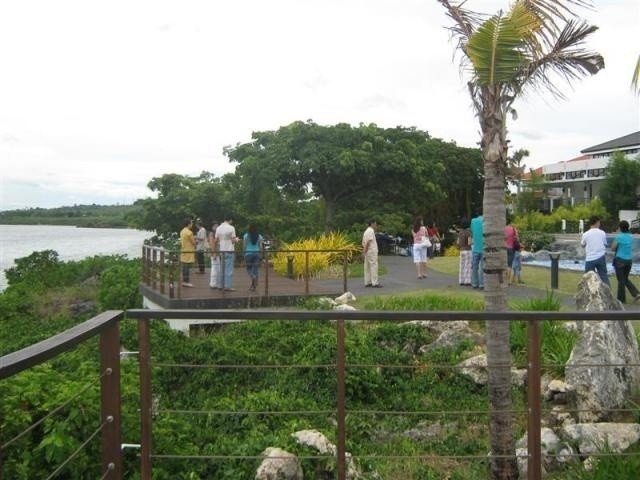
[364,284,384,288]
[632,292,640,303]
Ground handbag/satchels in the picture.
[513,241,521,250]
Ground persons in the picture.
[470,206,486,289]
[455,216,475,287]
[503,216,519,285]
[581,216,612,289]
[205,221,223,290]
[410,214,431,280]
[193,217,209,274]
[212,214,241,292]
[360,218,385,289]
[179,217,198,287]
[608,219,640,303]
[242,222,264,291]
[425,219,442,259]
[507,240,526,286]
